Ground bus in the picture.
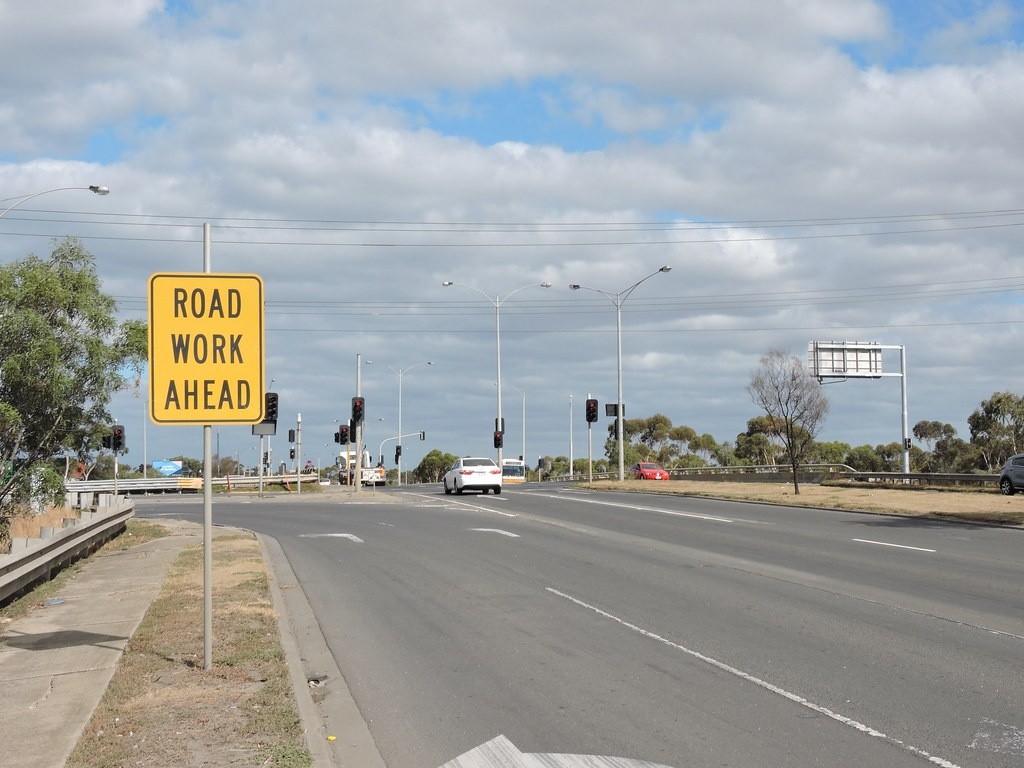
[495,458,527,483]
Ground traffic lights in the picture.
[264,452,268,461]
[351,396,365,423]
[264,392,279,421]
[494,431,504,448]
[396,446,401,455]
[102,433,111,448]
[111,425,125,451]
[290,448,296,459]
[263,458,266,465]
[339,425,349,444]
[139,464,143,473]
[585,399,599,422]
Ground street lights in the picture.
[443,281,552,471]
[365,359,435,486]
[569,264,673,482]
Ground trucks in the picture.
[335,451,387,486]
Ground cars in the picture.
[441,457,503,496]
[999,453,1024,496]
[319,477,341,486]
[628,462,671,481]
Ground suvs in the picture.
[223,474,259,489]
